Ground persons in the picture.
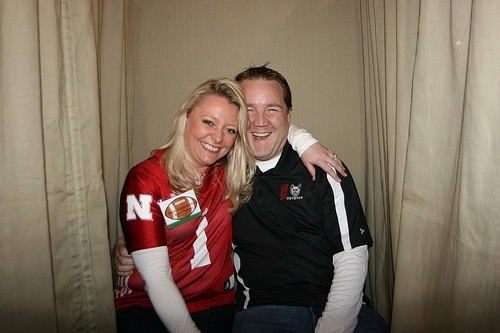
[111,77,348,333]
[110,66,392,333]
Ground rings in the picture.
[331,154,337,160]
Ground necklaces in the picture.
[195,171,206,186]
